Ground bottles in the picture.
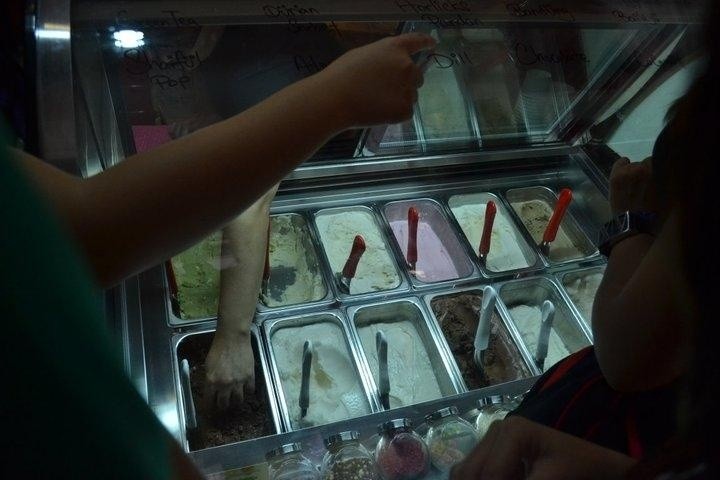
[263,389,532,479]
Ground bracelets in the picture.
[597,209,666,257]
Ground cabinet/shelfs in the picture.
[26,0,720,480]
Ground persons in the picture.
[0,32,438,480]
[117,24,280,409]
[448,0,720,392]
[450,248,720,480]
